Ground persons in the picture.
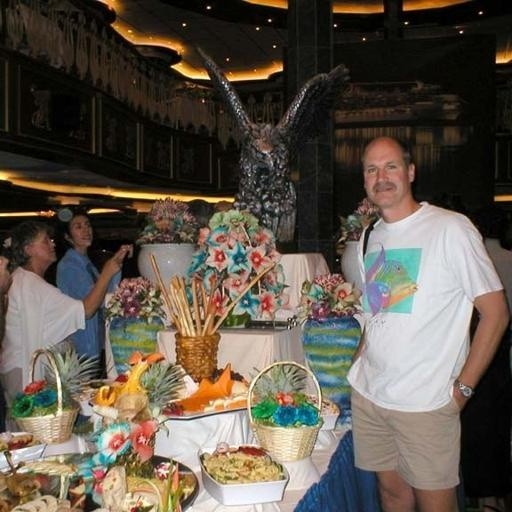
[1,220,126,434]
[346,134,512,512]
[0,254,13,296]
[54,203,134,386]
[451,203,511,511]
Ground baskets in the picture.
[16,350,82,444]
[175,332,222,380]
[246,360,324,464]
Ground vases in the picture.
[302,314,362,408]
[108,314,163,378]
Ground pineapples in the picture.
[29,344,100,416]
[134,364,184,426]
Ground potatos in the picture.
[0,470,36,512]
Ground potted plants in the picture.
[216,278,249,329]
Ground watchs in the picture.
[453,379,474,399]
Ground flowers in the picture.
[250,393,317,427]
[295,271,362,320]
[7,380,68,417]
[74,419,178,506]
[101,192,290,321]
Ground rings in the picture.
[127,246,129,248]
